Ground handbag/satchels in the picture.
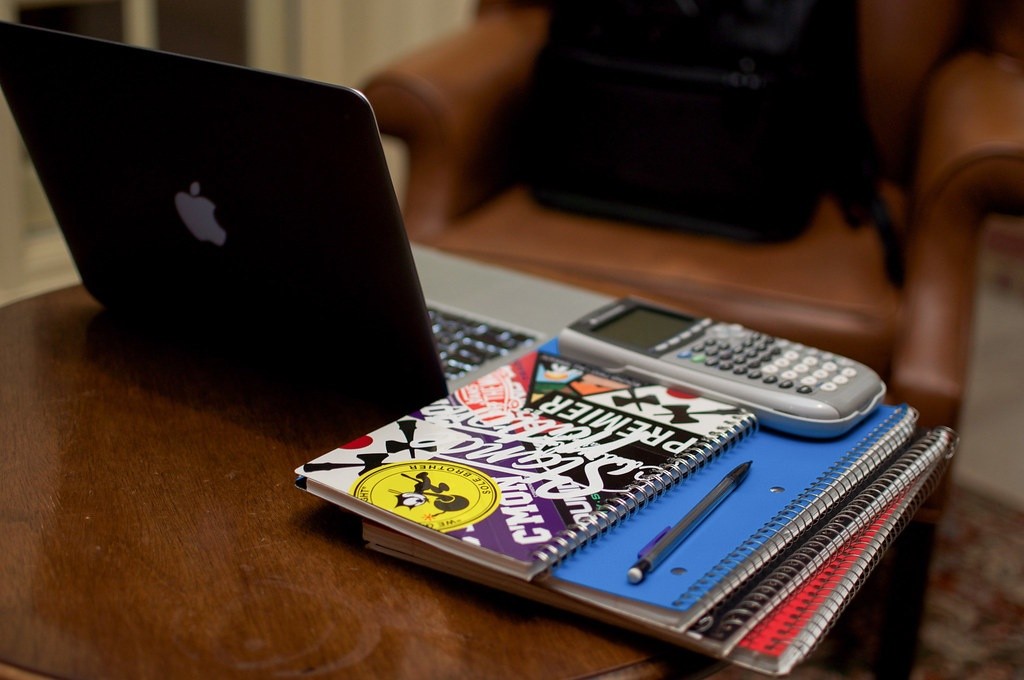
[521,0,906,291]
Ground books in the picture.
[290,337,962,676]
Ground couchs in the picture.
[358,0,1024,679]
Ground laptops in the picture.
[0,22,620,420]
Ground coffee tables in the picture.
[0,280,745,679]
